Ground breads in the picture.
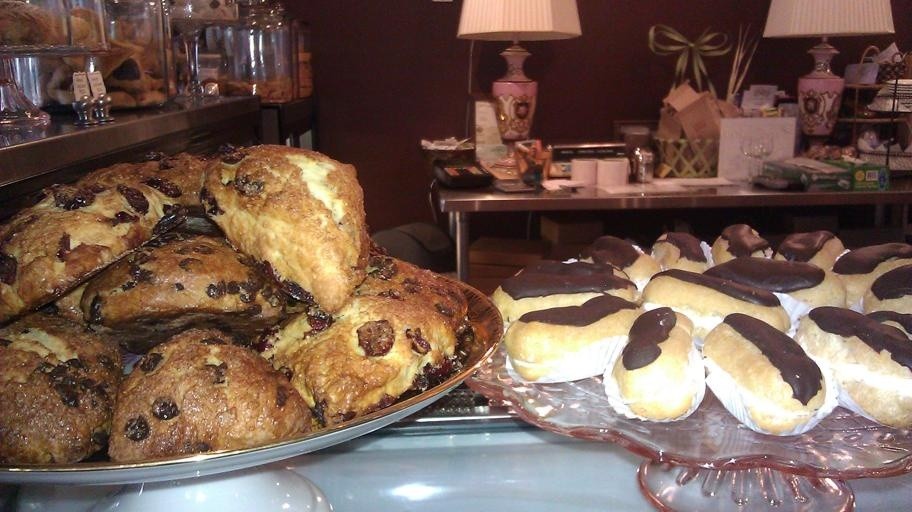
[1,0,240,109]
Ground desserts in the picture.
[490,222,912,436]
[0,142,476,464]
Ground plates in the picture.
[885,78,912,101]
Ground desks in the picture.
[439,170,910,283]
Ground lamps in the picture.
[454,0,581,144]
[760,0,895,136]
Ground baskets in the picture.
[651,135,718,180]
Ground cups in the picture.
[569,155,631,188]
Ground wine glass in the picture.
[738,134,774,184]
[1,275,506,512]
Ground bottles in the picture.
[16,0,182,119]
[217,0,315,105]
